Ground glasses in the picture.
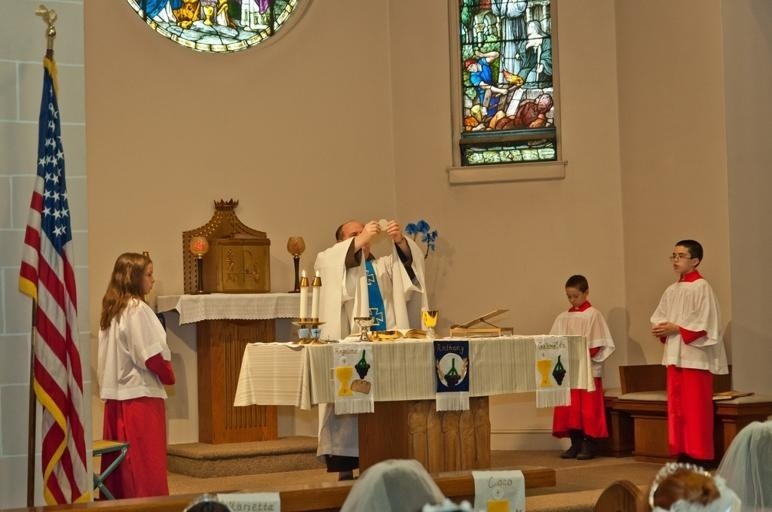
[668,254,694,261]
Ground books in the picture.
[712,389,755,401]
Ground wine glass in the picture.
[356,316,374,343]
[422,306,439,341]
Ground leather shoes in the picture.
[339,470,353,481]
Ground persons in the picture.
[716,415,771,512]
[94,250,179,502]
[643,461,742,512]
[466,48,509,120]
[190,0,223,35]
[307,218,435,481]
[482,93,553,131]
[338,457,452,512]
[514,21,552,87]
[645,238,732,471]
[546,271,618,460]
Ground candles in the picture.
[310,270,321,318]
[300,269,309,318]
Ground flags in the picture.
[16,3,89,508]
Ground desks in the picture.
[233,335,596,479]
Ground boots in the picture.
[561,431,583,458]
[577,436,594,460]
